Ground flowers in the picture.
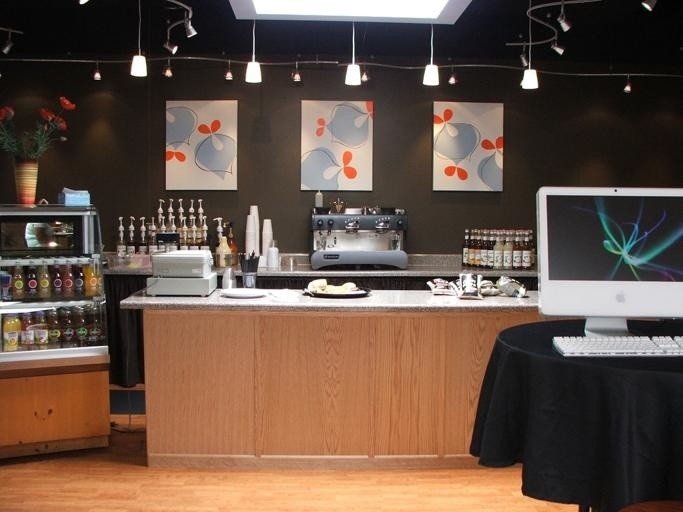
[0,96,76,161]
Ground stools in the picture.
[617,500,683,512]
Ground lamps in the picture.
[184,18,197,38]
[551,40,566,56]
[1,27,25,55]
[640,0,657,12]
[291,63,301,82]
[130,2,150,77]
[519,1,539,92]
[163,58,174,78]
[93,62,102,81]
[242,19,265,85]
[418,24,442,87]
[447,66,457,85]
[622,73,632,91]
[362,65,371,83]
[342,20,363,86]
[557,5,573,34]
[163,41,178,55]
[225,61,234,81]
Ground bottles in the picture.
[315,189,323,207]
[461,228,536,270]
[3,260,104,353]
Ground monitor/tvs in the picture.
[536,186,683,335]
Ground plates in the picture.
[220,287,266,297]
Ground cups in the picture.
[243,272,257,288]
[245,205,272,255]
[268,247,279,268]
[222,267,237,287]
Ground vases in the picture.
[14,161,39,207]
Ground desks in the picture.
[467,320,683,512]
[118,287,542,474]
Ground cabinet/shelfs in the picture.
[0,204,117,459]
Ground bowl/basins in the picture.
[315,207,331,214]
[281,256,296,266]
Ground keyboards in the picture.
[551,335,683,357]
